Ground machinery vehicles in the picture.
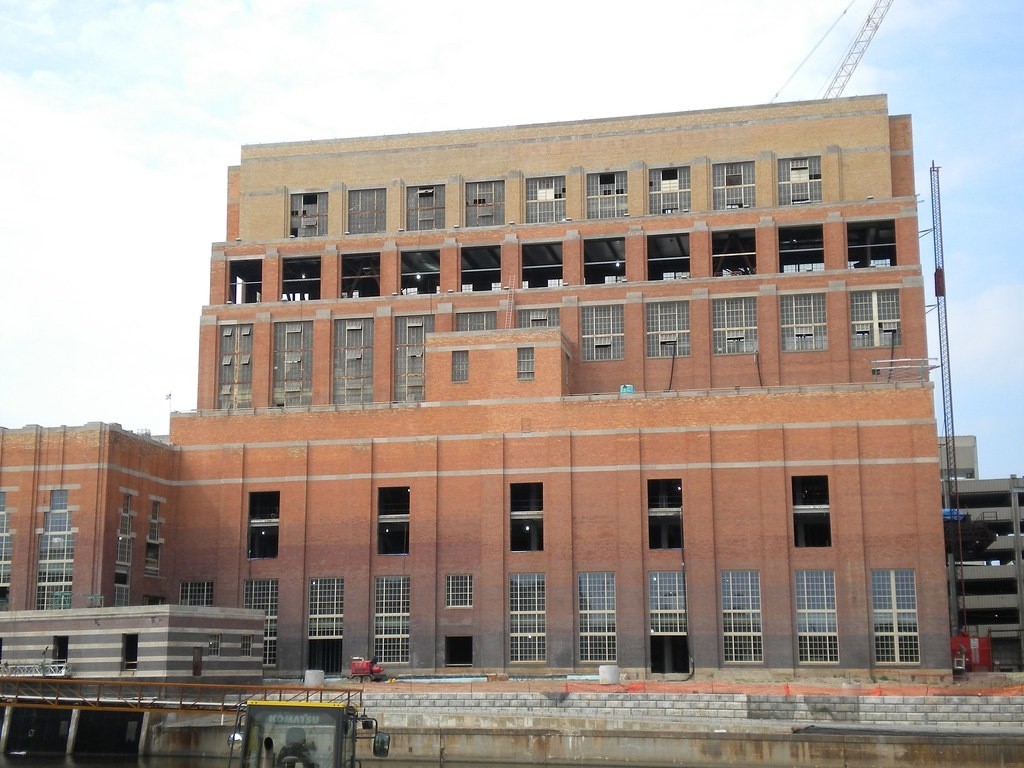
[351,657,386,683]
[229,699,390,768]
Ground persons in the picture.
[278,728,319,768]
[992,656,1000,672]
[377,739,387,756]
